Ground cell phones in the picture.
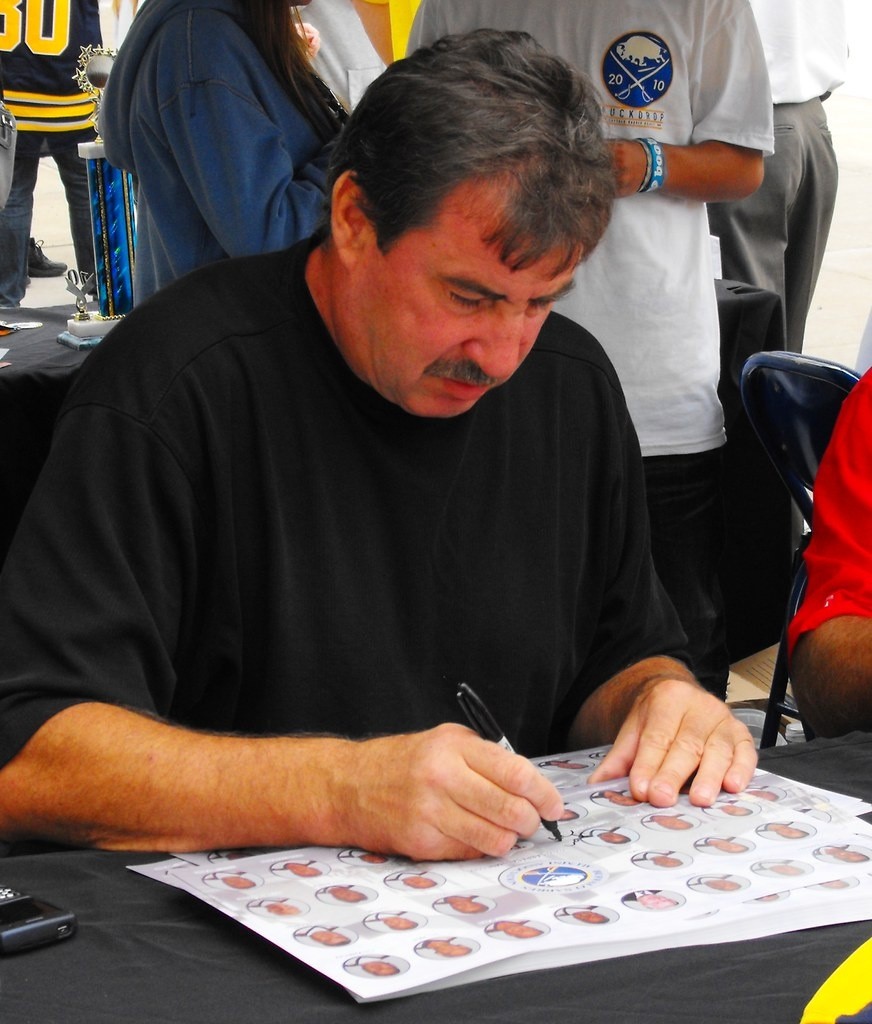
[0,884,79,955]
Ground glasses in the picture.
[309,69,351,131]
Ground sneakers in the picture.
[27,237,67,276]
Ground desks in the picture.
[0,735,872,1024]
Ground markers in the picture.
[455,677,563,845]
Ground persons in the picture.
[97,0,341,307]
[787,367,872,739]
[0,27,760,861]
[404,0,775,703]
[0,0,103,309]
[705,0,848,354]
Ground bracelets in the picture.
[635,136,663,193]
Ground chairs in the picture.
[739,351,862,749]
